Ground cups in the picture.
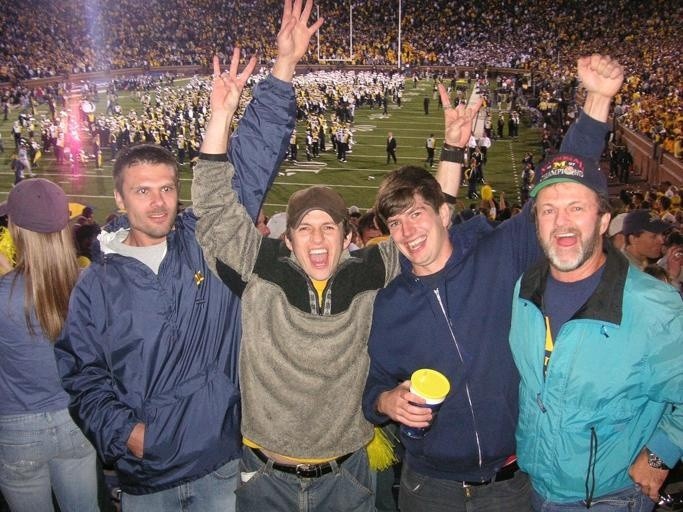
[403,368,450,438]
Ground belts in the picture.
[252,447,348,478]
[447,462,521,487]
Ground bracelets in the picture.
[645,450,669,471]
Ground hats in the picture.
[608,208,674,237]
[529,153,608,199]
[265,213,287,240]
[1,179,71,233]
[287,186,349,228]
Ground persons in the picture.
[1,179,101,512]
[191,46,486,511]
[53,0,326,511]
[362,54,626,512]
[509,155,683,512]
[2,1,681,311]
